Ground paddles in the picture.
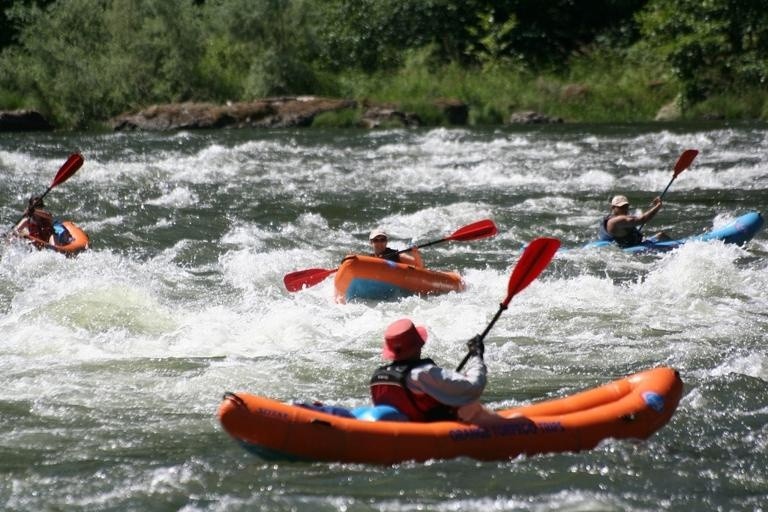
[10,155,85,230]
[285,219,498,293]
[456,237,562,374]
[637,150,697,231]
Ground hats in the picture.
[369,230,388,239]
[30,197,44,207]
[612,195,629,207]
[382,319,427,359]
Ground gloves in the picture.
[468,336,484,359]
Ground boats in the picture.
[214,363,689,469]
[12,219,97,258]
[333,250,470,307]
[530,211,763,271]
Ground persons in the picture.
[13,196,57,250]
[599,195,674,248]
[359,229,424,269]
[370,318,531,423]
